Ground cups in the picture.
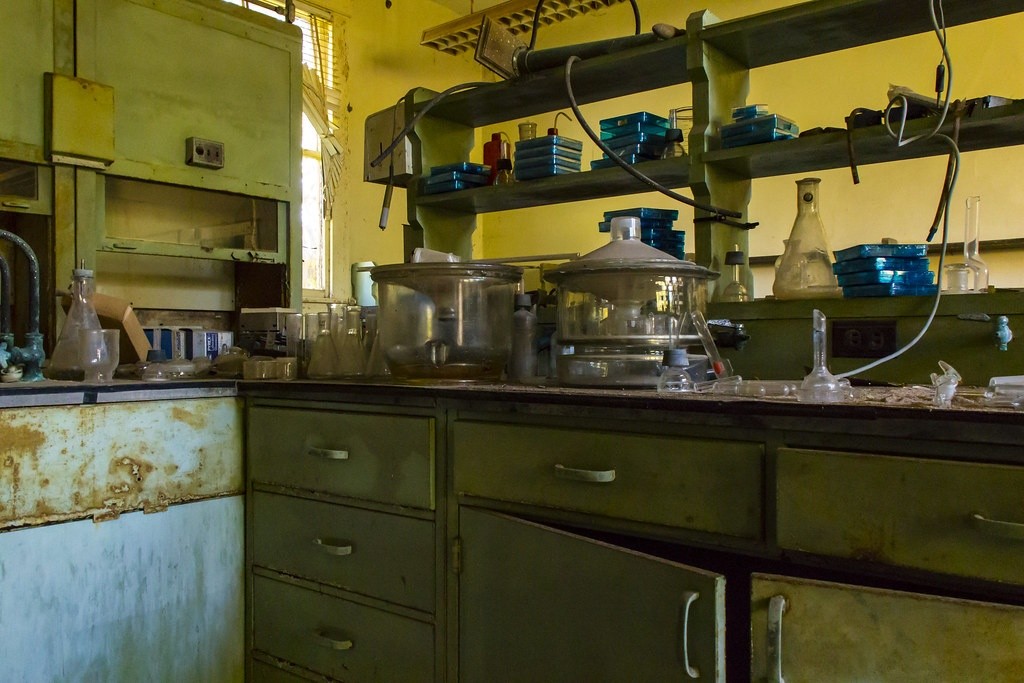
[77,329,120,384]
[675,107,693,156]
[243,357,297,379]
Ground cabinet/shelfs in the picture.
[1,0,303,682]
[243,395,1023,682]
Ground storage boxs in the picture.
[180,327,235,359]
[718,103,801,147]
[515,136,585,181]
[832,244,938,299]
[422,162,493,195]
[61,289,152,364]
[143,326,186,359]
[590,112,670,172]
[598,208,684,261]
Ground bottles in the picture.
[659,109,684,159]
[47,268,110,368]
[285,299,392,380]
[656,317,692,390]
[512,121,537,183]
[483,132,510,186]
[774,239,808,292]
[493,140,515,186]
[723,244,749,302]
[797,308,843,402]
[773,178,844,299]
[137,327,172,381]
